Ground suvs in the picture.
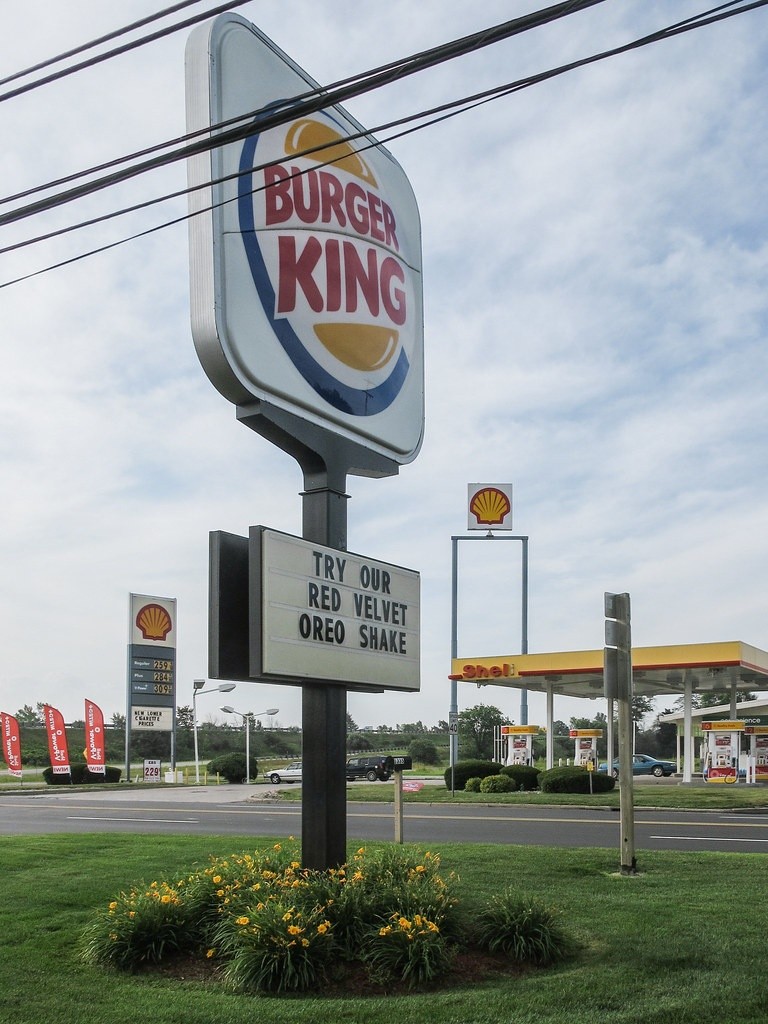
[346,756,385,782]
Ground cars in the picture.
[264,761,302,784]
[597,753,677,780]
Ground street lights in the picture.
[220,705,280,784]
[192,679,237,786]
